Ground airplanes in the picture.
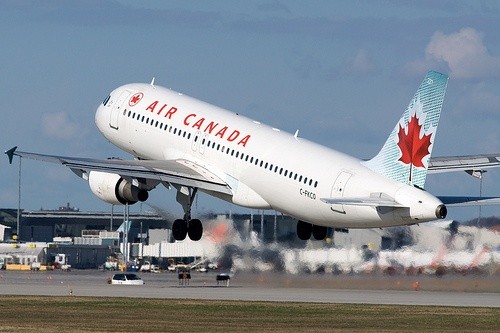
[6,68,500,248]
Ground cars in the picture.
[112,272,145,287]
